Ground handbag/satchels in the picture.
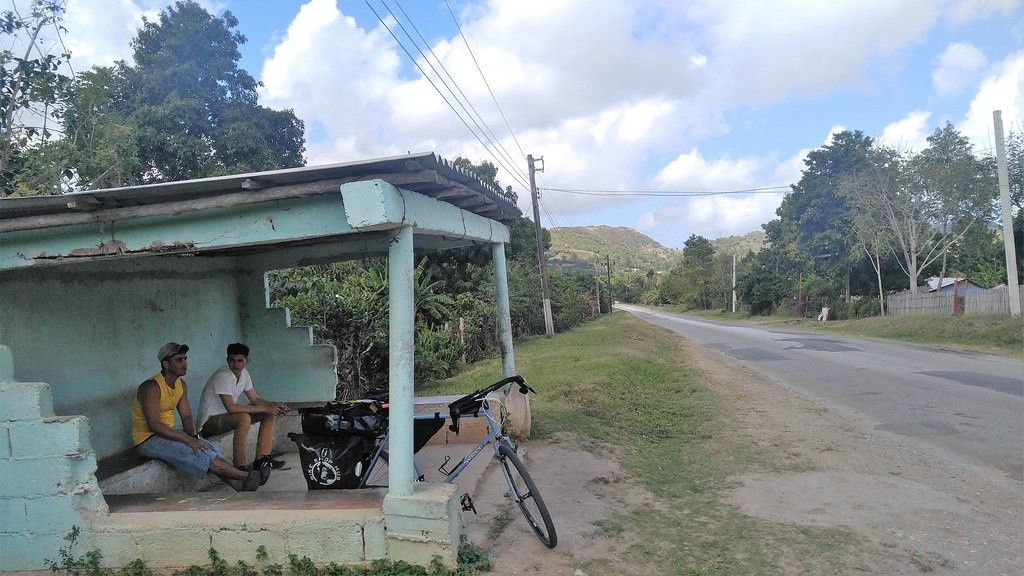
[287,430,377,490]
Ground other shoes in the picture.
[242,468,270,492]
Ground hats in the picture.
[158,343,189,365]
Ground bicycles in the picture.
[288,372,558,549]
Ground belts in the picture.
[135,434,157,447]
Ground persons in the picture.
[131,342,272,493]
[197,344,290,472]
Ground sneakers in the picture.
[237,466,249,471]
[254,456,286,472]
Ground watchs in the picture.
[192,433,201,439]
